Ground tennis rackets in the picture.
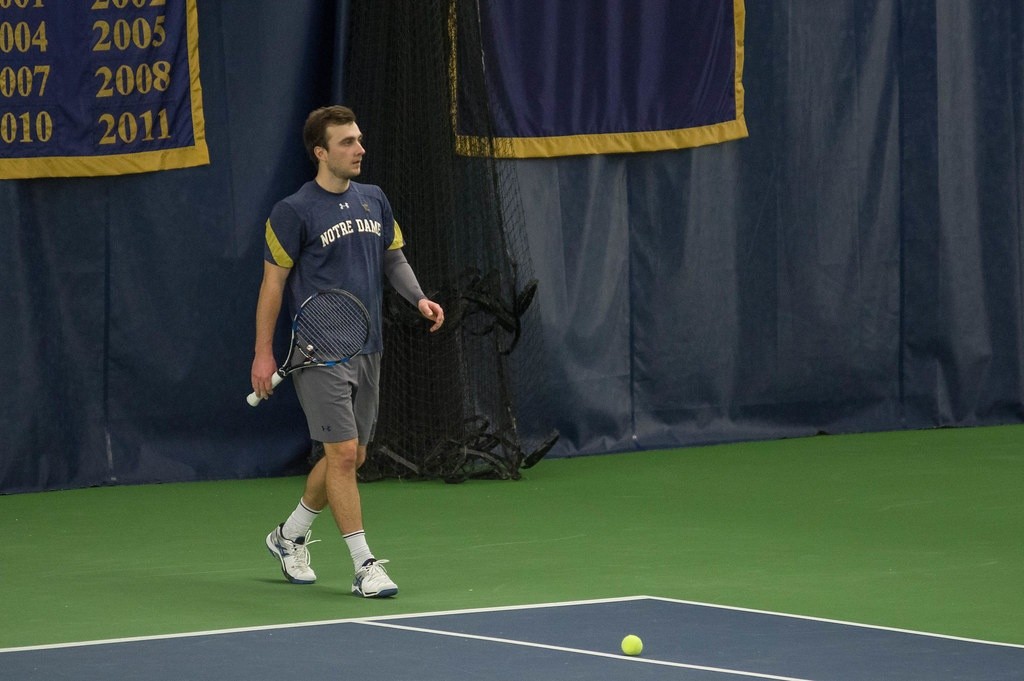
[246,288,372,407]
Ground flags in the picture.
[445,0,751,159]
[0,0,210,184]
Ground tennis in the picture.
[621,635,643,656]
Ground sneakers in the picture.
[265,522,317,583]
[351,557,399,597]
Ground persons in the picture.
[249,106,445,598]
